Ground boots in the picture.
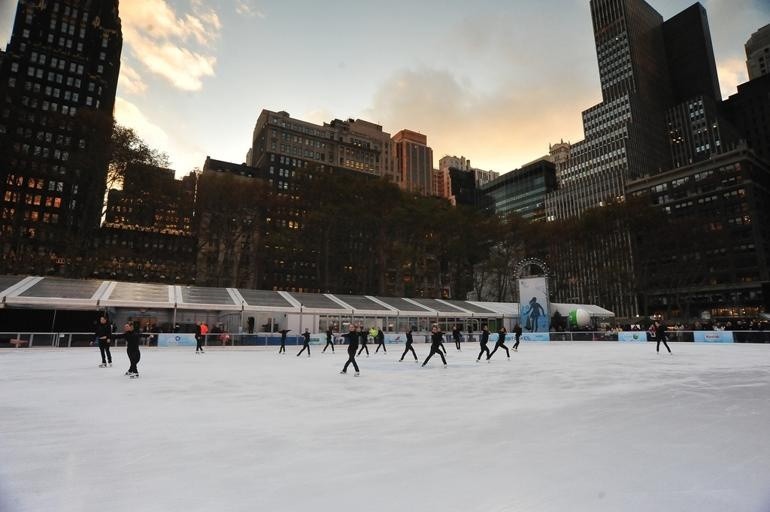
[125,371,139,377]
[99,362,112,366]
[279,348,523,376]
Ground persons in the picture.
[89,317,229,377]
[522,297,546,333]
